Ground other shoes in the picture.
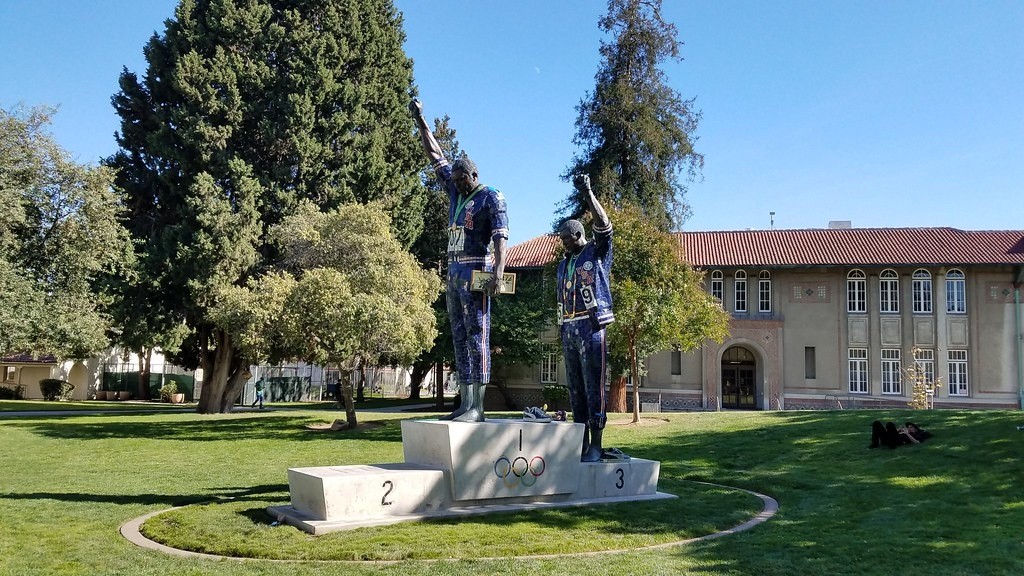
[868,440,881,448]
[887,441,896,449]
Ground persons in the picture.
[408,98,509,422]
[556,174,617,463]
[868,420,925,449]
[552,409,568,423]
[335,379,342,404]
[252,377,266,409]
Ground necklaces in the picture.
[563,265,576,318]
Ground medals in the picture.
[567,281,572,288]
[452,222,456,231]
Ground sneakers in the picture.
[601,447,631,462]
[523,407,552,423]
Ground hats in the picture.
[905,421,918,429]
